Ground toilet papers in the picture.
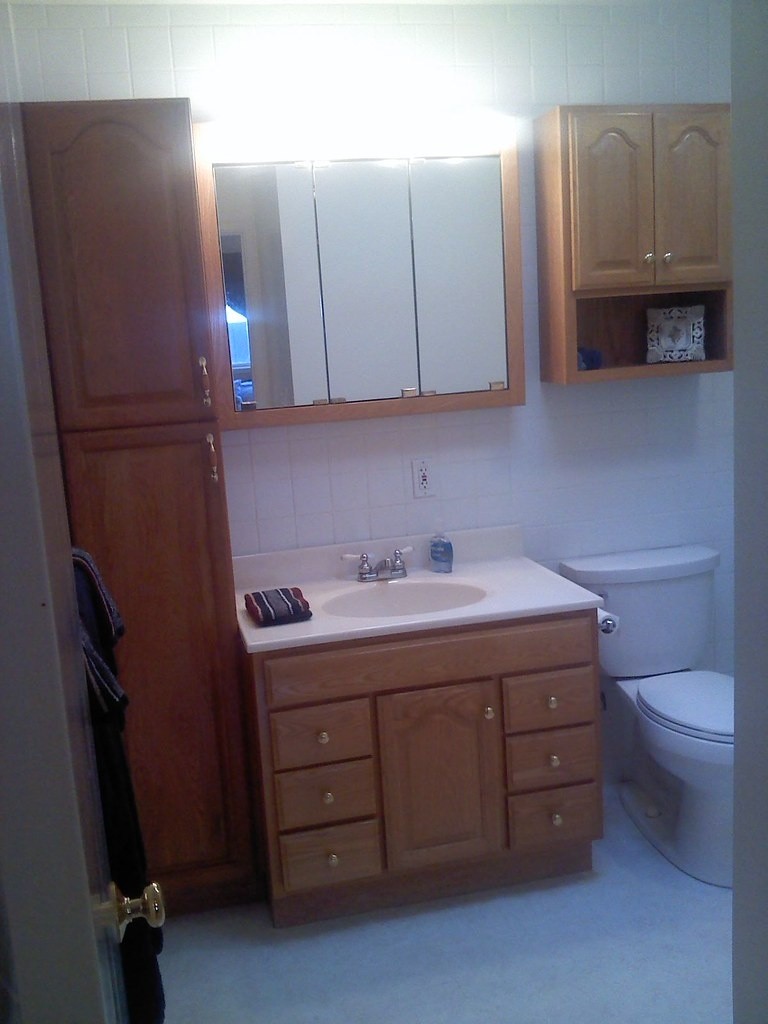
[597,607,620,637]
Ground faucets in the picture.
[372,556,396,581]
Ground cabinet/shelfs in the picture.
[21,97,265,919]
[532,103,734,387]
[236,607,603,930]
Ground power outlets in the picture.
[411,458,436,499]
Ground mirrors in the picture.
[197,150,526,432]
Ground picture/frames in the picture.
[646,304,705,363]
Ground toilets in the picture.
[558,544,733,890]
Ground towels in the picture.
[243,586,313,627]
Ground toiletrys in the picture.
[428,528,454,573]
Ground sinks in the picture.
[318,569,488,636]
